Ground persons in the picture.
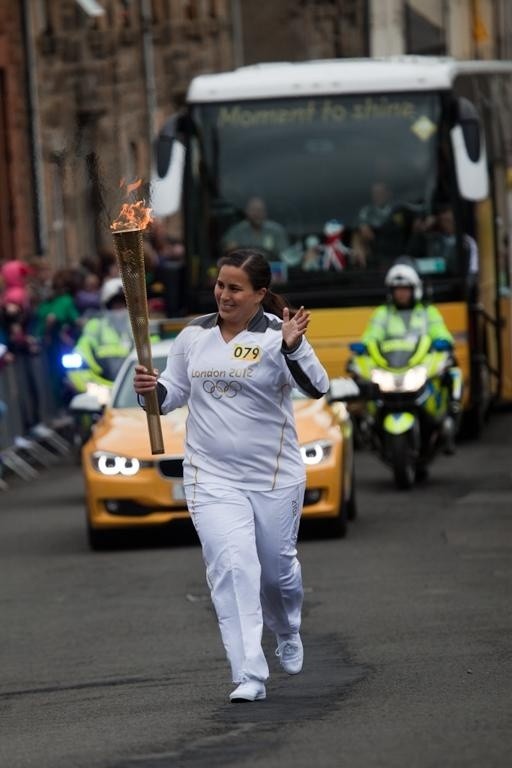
[1,228,187,451]
[421,206,482,299]
[351,264,461,460]
[357,182,411,268]
[131,248,332,703]
[224,196,291,259]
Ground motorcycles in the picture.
[340,326,467,490]
[55,319,161,447]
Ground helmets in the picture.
[385,264,424,299]
[102,279,127,316]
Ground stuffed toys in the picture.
[319,218,355,272]
[298,234,322,274]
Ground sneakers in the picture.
[273,631,304,674]
[230,681,266,702]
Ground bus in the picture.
[136,55,511,438]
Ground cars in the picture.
[76,318,358,552]
